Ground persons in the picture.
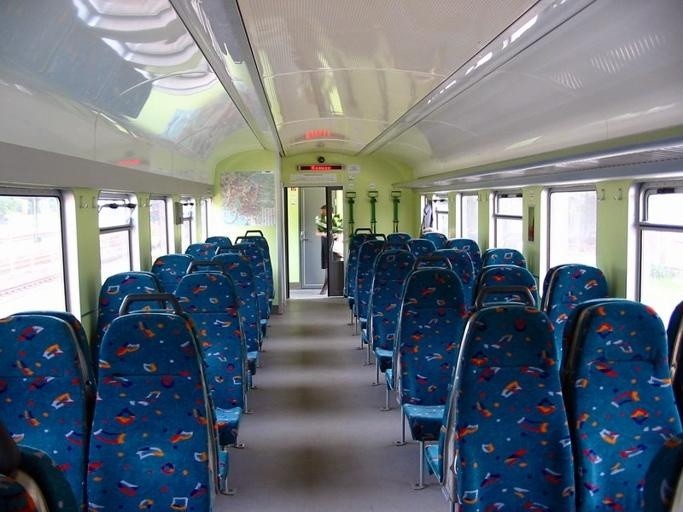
[315,205,338,295]
[641,432,683,512]
[0,424,56,512]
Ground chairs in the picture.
[343,228,683,512]
[0,231,275,512]
